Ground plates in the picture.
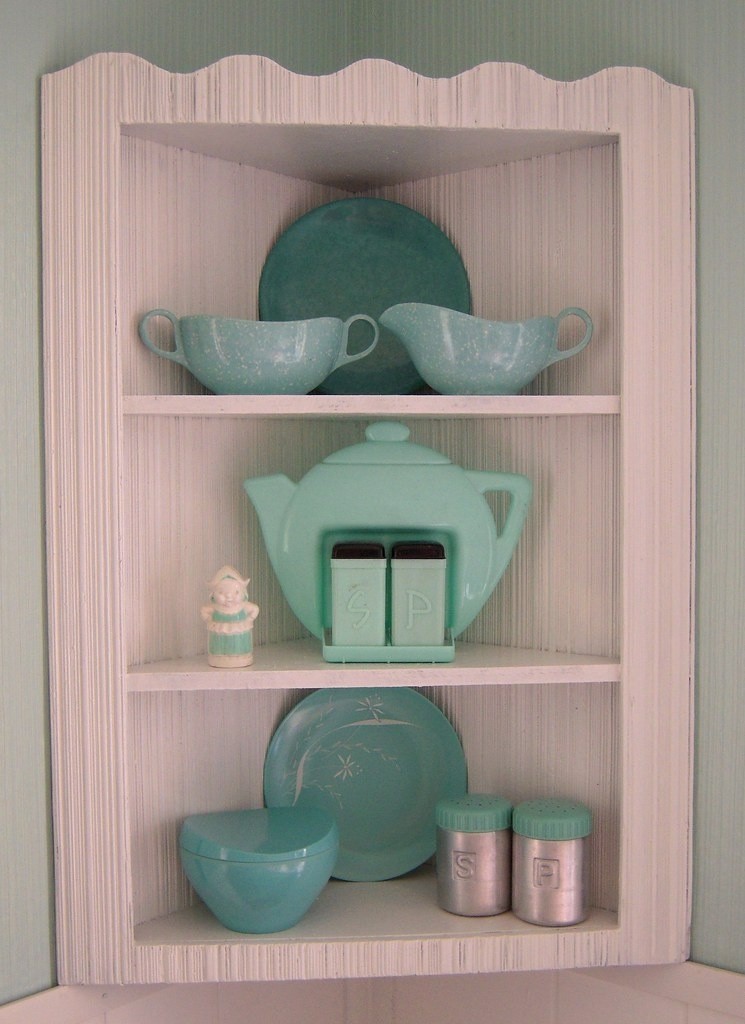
[263,688,468,881]
[260,197,471,395]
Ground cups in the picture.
[380,303,592,395]
[139,309,379,395]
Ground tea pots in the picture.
[243,421,531,663]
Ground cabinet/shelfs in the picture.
[40,54,694,986]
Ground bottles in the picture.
[436,795,512,917]
[512,798,592,927]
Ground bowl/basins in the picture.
[180,808,339,935]
